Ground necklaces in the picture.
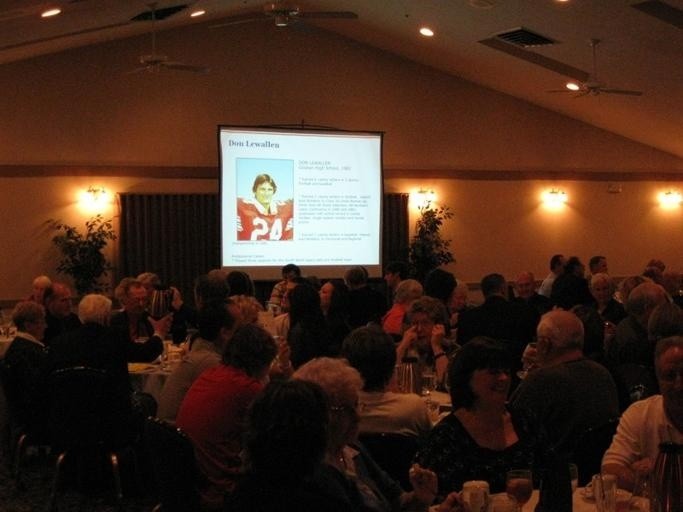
[330,446,345,465]
[461,406,497,436]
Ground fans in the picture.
[207,1,361,30]
[542,36,646,101]
[116,1,204,78]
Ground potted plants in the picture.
[45,214,117,303]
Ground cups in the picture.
[161,342,176,373]
[487,493,518,512]
[463,480,489,512]
[592,474,618,512]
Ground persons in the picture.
[14,275,52,304]
[236,172,295,242]
[507,310,626,512]
[42,282,81,339]
[422,268,456,305]
[287,284,336,372]
[318,279,346,333]
[424,338,542,499]
[383,279,423,335]
[581,272,626,363]
[176,323,278,512]
[600,336,683,512]
[383,261,411,298]
[539,254,565,297]
[112,278,161,339]
[617,272,652,312]
[270,264,301,317]
[226,270,264,329]
[456,272,530,347]
[395,297,454,388]
[642,266,662,286]
[225,379,362,511]
[551,256,599,311]
[628,303,683,401]
[510,271,552,346]
[273,278,321,335]
[328,266,390,336]
[0,301,67,447]
[53,293,172,465]
[158,303,236,418]
[585,255,608,280]
[291,355,436,511]
[137,272,160,297]
[646,257,665,272]
[602,282,669,397]
[343,324,434,437]
[167,273,231,329]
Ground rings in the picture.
[605,323,609,326]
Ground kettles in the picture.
[650,441,683,512]
[151,283,168,321]
[401,358,416,396]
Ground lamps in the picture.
[74,185,111,223]
[659,188,683,214]
[408,183,435,210]
[540,186,568,214]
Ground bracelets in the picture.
[432,351,447,360]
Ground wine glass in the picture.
[421,372,439,404]
[443,371,451,403]
[570,463,579,492]
[506,468,533,512]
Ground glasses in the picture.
[479,365,513,377]
[532,334,543,340]
[329,401,368,416]
